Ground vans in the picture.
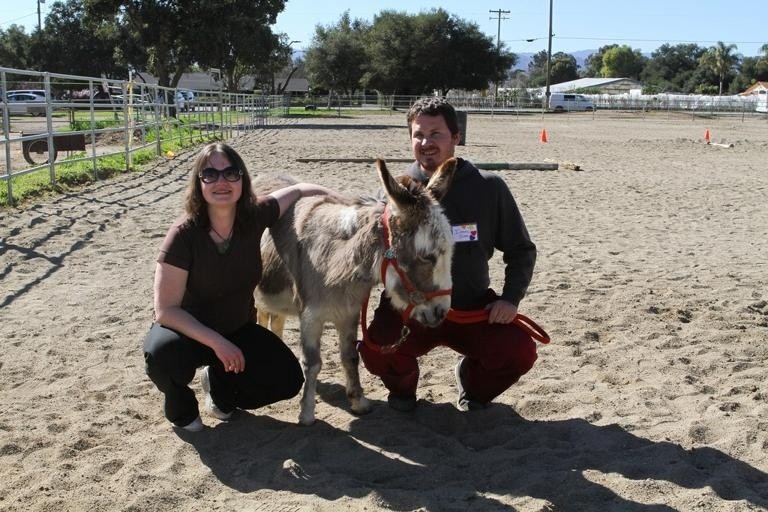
[549,95,594,111]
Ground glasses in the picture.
[201,166,240,183]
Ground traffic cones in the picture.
[540,127,547,143]
[705,128,711,143]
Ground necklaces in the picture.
[210,225,233,250]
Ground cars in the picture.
[93,90,195,112]
[1,86,55,116]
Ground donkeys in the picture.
[238,154,460,429]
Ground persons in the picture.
[358,97,538,414]
[143,143,349,433]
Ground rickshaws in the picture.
[20,132,85,166]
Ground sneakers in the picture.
[169,416,204,432]
[197,366,234,421]
[454,357,482,413]
[386,388,416,411]
